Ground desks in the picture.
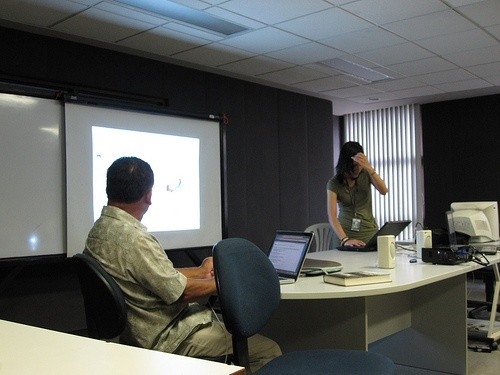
[279,244,500,375]
[0,319,246,375]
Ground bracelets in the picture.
[370,170,376,175]
[340,236,349,246]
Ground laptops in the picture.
[267,230,314,285]
[336,220,411,252]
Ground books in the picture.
[322,270,393,286]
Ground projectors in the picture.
[421,245,476,266]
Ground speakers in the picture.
[377,235,396,269]
[416,229,433,258]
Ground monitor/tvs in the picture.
[446,200,500,254]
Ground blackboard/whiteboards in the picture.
[0,75,67,265]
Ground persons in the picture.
[326,141,388,246]
[82,156,282,375]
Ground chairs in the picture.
[301,223,336,250]
[74,253,144,347]
[212,239,396,375]
